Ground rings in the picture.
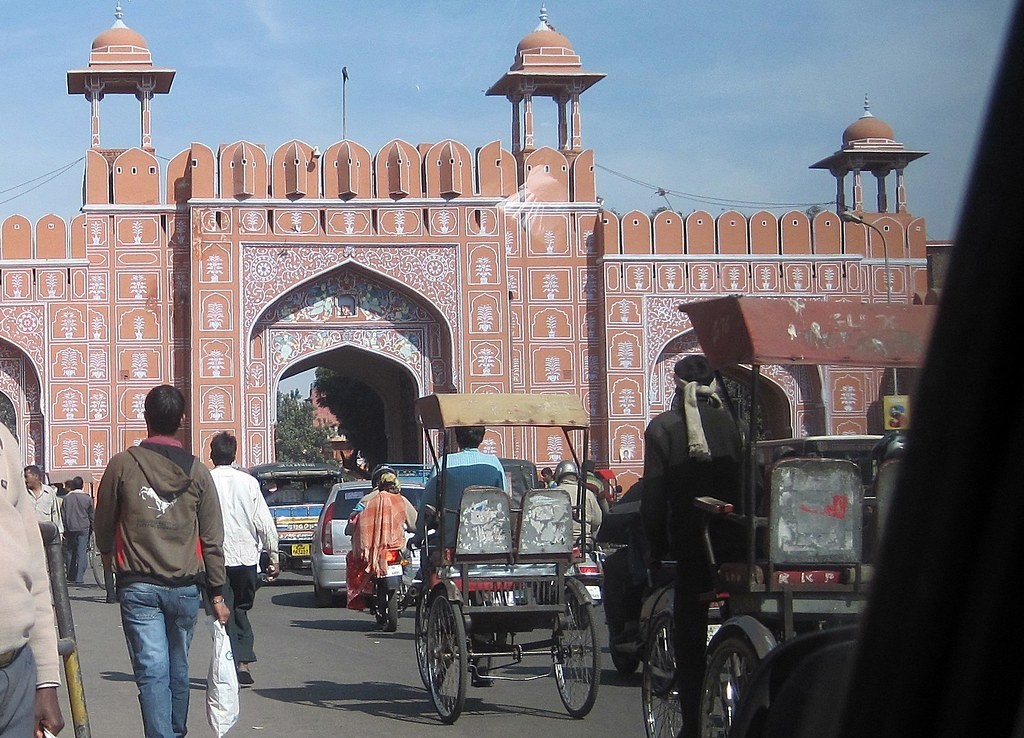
[222,621,225,624]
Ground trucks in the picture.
[252,463,346,569]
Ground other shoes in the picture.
[614,623,642,645]
[238,668,255,687]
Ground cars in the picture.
[496,459,541,510]
[308,481,436,607]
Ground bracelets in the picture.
[211,599,225,603]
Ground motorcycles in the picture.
[574,535,602,632]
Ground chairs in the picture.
[870,434,910,584]
[717,458,863,599]
[515,487,573,563]
[454,485,514,565]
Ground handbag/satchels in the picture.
[205,620,240,738]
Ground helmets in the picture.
[554,461,579,486]
[372,464,397,488]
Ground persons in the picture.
[538,460,609,543]
[344,465,420,610]
[0,421,94,738]
[94,384,230,738]
[407,427,509,687]
[638,355,745,738]
[210,432,279,686]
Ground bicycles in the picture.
[88,531,117,590]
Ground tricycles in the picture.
[641,295,939,738]
[409,394,603,726]
